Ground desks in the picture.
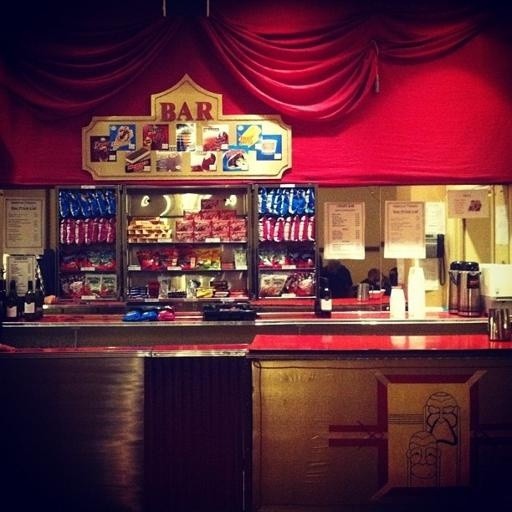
[331,293,389,311]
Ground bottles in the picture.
[314,272,332,318]
[5,279,43,321]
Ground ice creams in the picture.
[113,128,130,151]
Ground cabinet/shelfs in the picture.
[251,183,322,300]
[53,185,121,302]
[120,185,256,303]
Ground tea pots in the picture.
[449,261,482,316]
[488,308,511,341]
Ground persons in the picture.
[382,267,398,297]
[322,259,353,298]
[359,267,381,291]
